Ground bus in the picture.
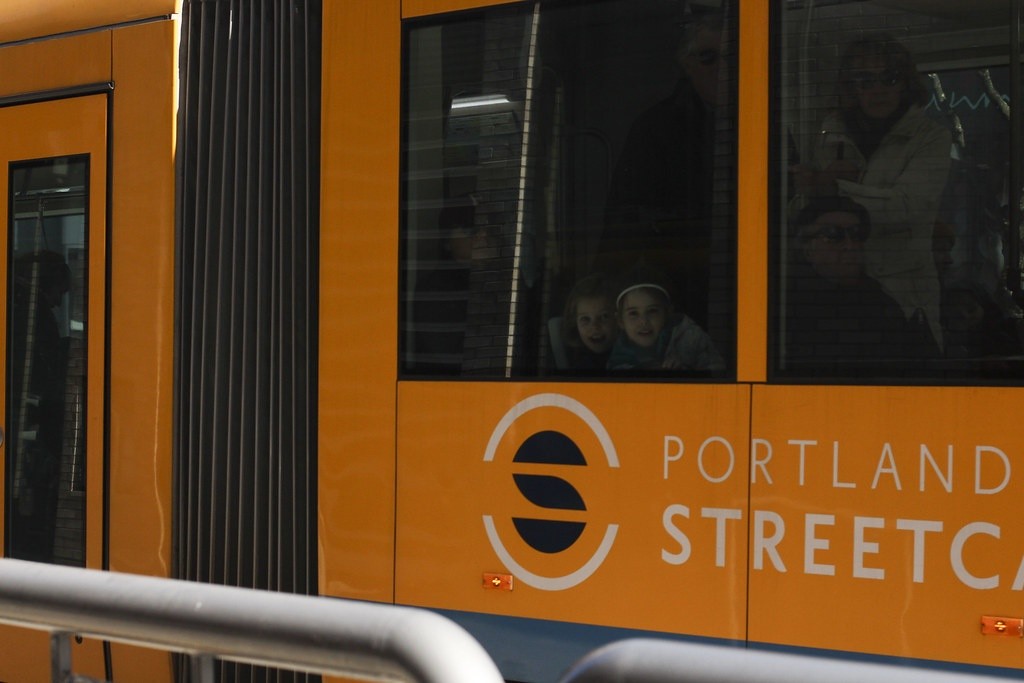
[0,0,1024,683]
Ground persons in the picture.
[591,15,801,334]
[11,248,71,537]
[412,181,529,375]
[557,274,626,381]
[787,32,1024,378]
[608,266,728,379]
